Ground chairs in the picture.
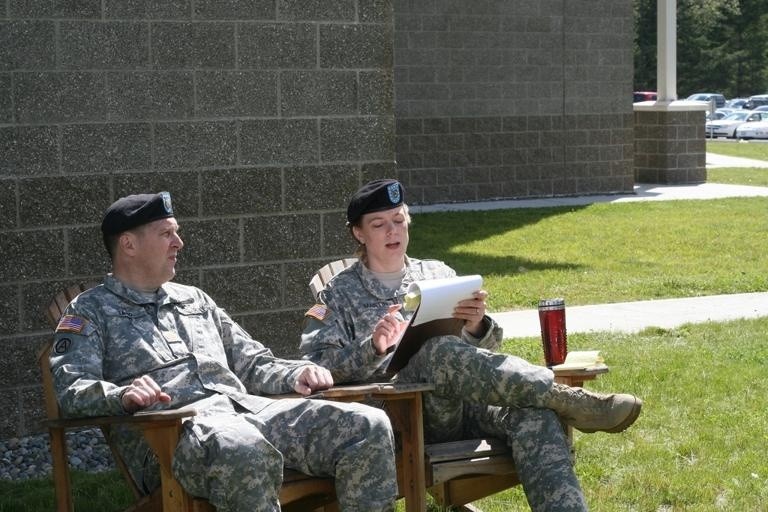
[39,282,394,512]
[309,258,608,512]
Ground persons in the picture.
[297,177,643,511]
[48,191,400,512]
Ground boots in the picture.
[542,381,643,434]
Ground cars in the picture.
[633,91,768,139]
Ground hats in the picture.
[347,179,405,217]
[101,190,175,239]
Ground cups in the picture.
[537,296,569,368]
